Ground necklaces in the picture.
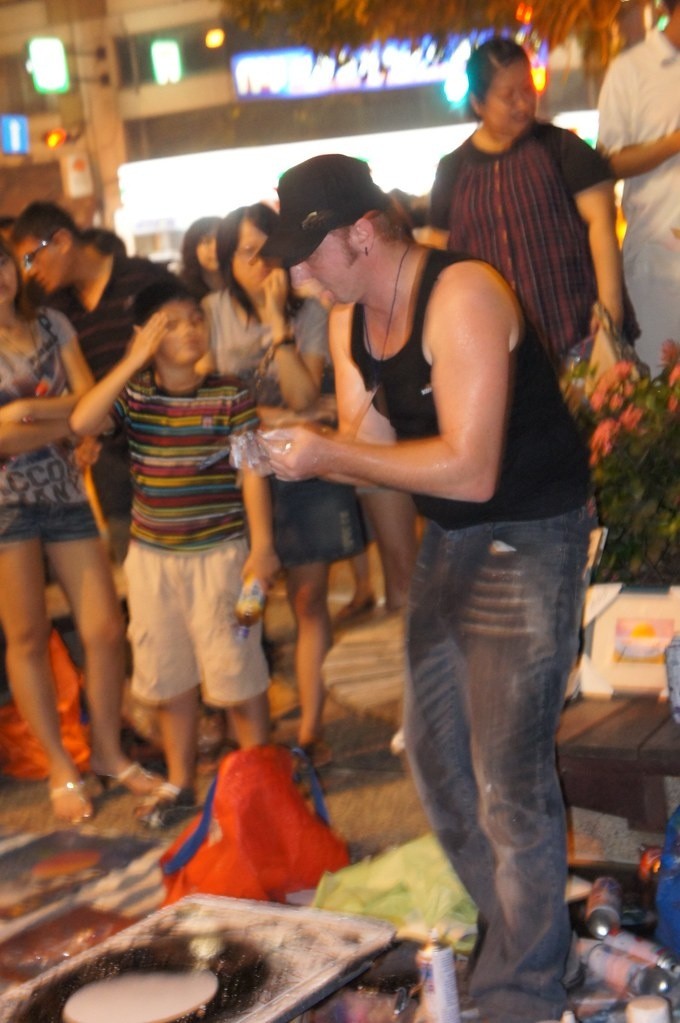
[364,243,410,365]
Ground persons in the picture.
[69,279,283,829]
[0,199,191,823]
[417,36,626,378]
[594,0,680,381]
[177,154,599,1023]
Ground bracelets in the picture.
[261,331,297,347]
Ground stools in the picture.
[553,694,680,831]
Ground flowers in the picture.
[564,340,680,584]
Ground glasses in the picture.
[23,230,57,267]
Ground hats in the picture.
[250,154,377,268]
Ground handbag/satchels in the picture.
[586,300,649,401]
[158,744,350,910]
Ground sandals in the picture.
[50,780,93,826]
[93,760,162,794]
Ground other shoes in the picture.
[136,784,196,824]
[338,594,373,621]
